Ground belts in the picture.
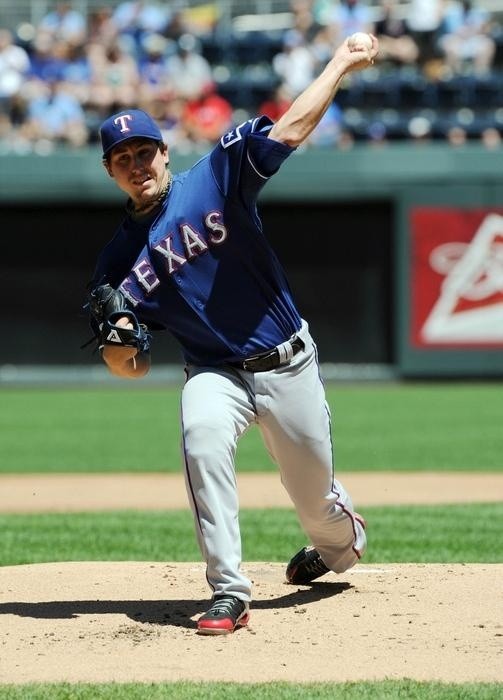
[191,338,303,372]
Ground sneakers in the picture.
[196,596,250,635]
[286,512,364,585]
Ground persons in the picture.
[87,32,380,635]
[81,32,380,635]
[1,3,502,144]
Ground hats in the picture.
[99,109,161,159]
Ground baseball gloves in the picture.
[82,282,152,356]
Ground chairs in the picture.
[0,0,503,148]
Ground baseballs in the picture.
[348,31,373,53]
